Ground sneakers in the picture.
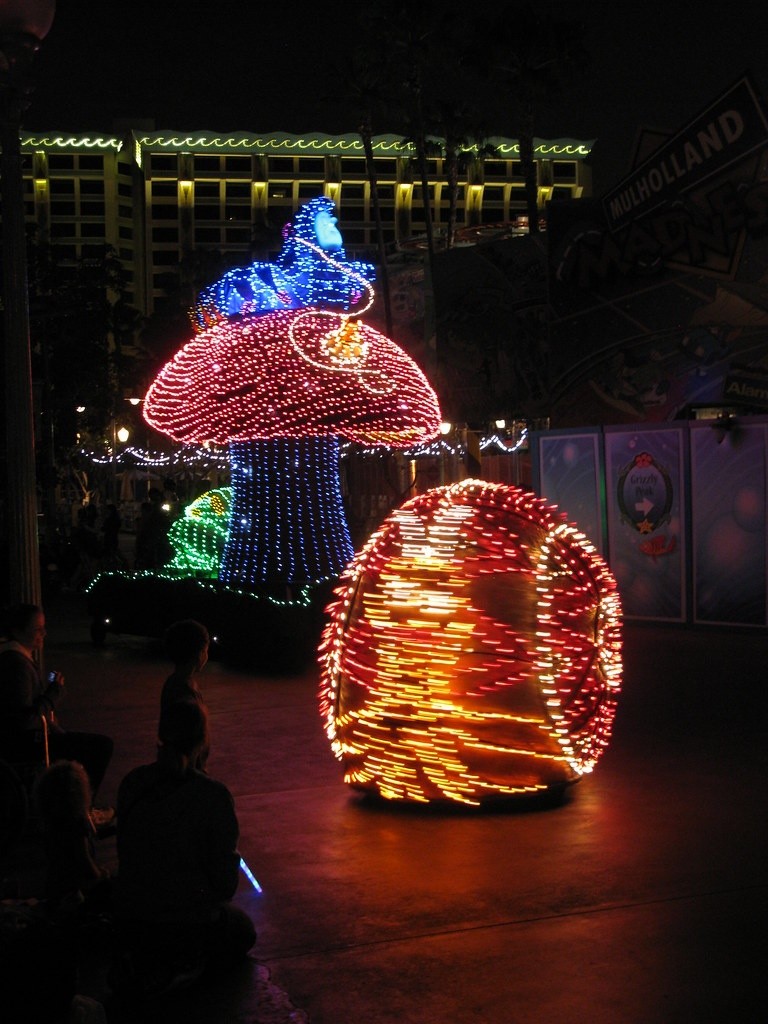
[83,807,115,828]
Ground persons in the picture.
[0,603,259,1024]
[138,478,179,561]
[77,504,121,549]
